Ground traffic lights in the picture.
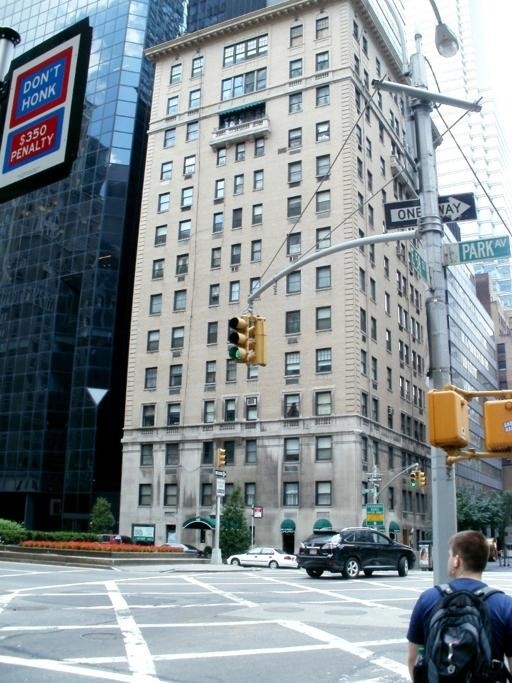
[419,471,426,487]
[246,316,266,366]
[226,314,248,363]
[409,472,416,487]
[216,446,227,467]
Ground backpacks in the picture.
[412,584,505,681]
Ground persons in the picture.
[405,529,512,683]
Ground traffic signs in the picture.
[214,469,227,478]
[382,190,477,226]
[444,235,509,264]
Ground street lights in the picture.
[379,0,463,584]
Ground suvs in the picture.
[298,525,417,578]
[98,533,137,546]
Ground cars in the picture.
[153,543,208,561]
[227,547,300,570]
